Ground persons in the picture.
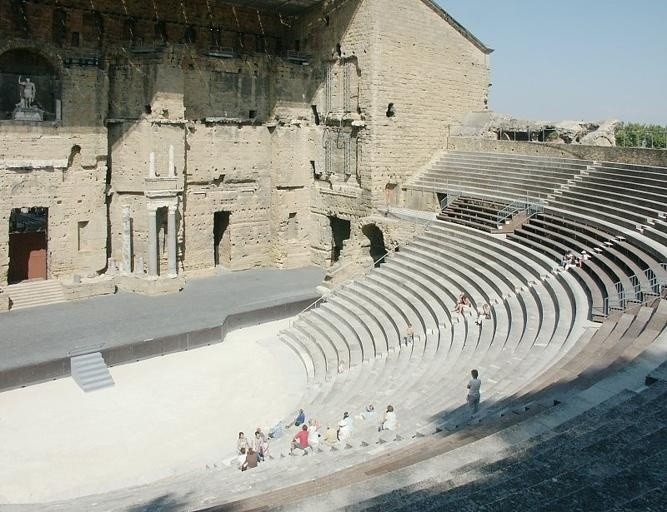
[18,75,36,109]
[466,370,481,417]
[338,359,344,374]
[451,290,493,326]
[377,405,400,433]
[234,402,375,474]
[405,322,414,345]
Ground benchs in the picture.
[193,406,552,512]
[435,193,666,410]
